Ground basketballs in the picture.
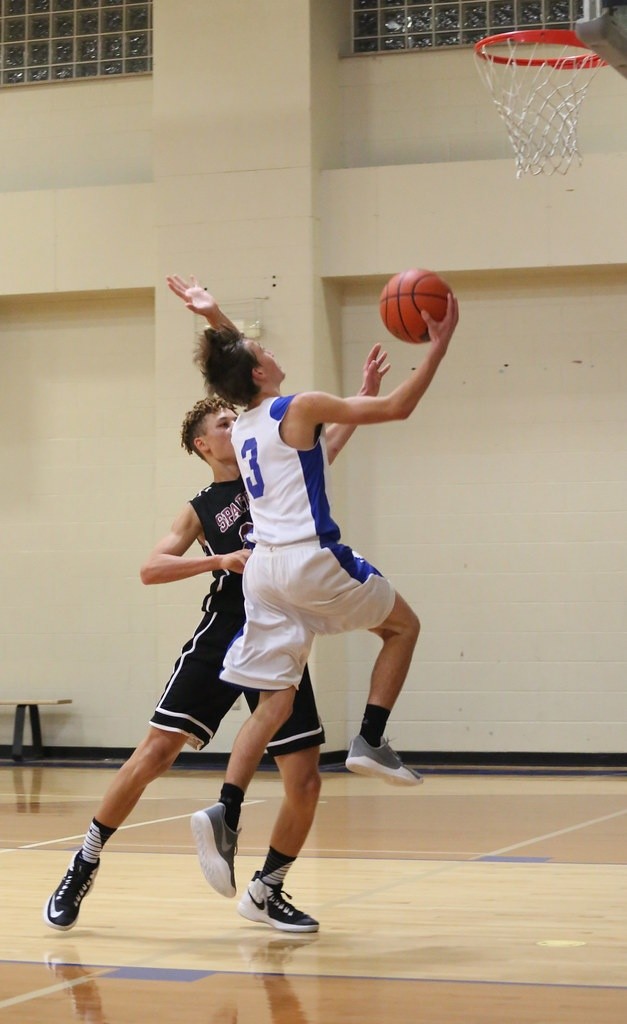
[379,267,452,345]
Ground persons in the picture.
[169,274,459,899]
[46,343,390,933]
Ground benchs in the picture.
[1,699,70,759]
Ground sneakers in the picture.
[240,870,320,931]
[192,802,239,897]
[345,734,424,784]
[45,850,101,931]
